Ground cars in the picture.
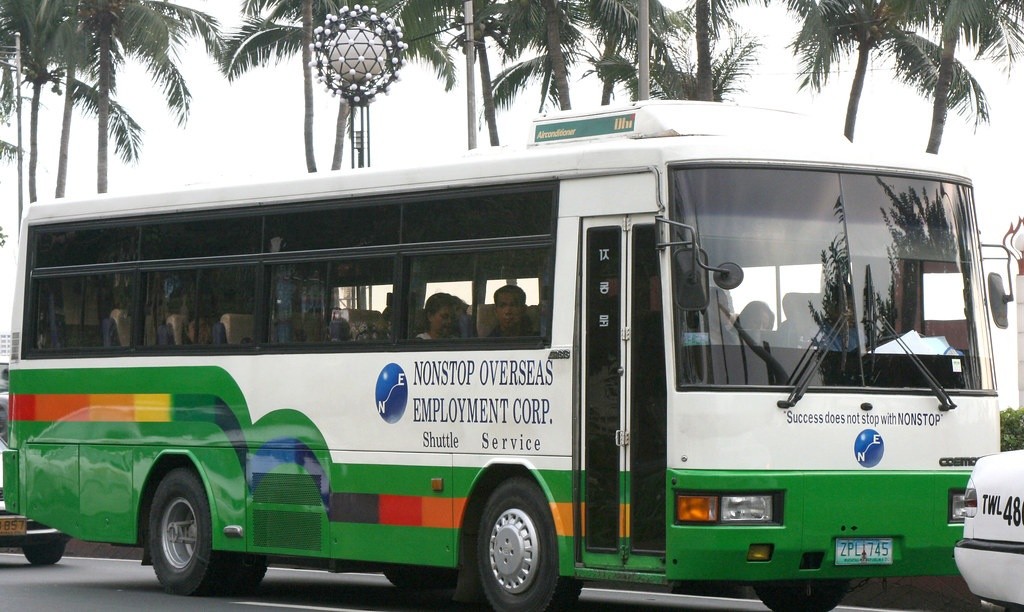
[0,394,71,561]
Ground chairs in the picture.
[49,304,544,350]
[783,292,826,340]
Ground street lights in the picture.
[317,5,407,170]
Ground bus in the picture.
[3,98,1020,612]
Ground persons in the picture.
[327,318,350,342]
[415,292,469,339]
[184,313,214,344]
[777,293,805,370]
[730,301,776,386]
[486,285,527,337]
[813,281,869,353]
[705,286,743,386]
